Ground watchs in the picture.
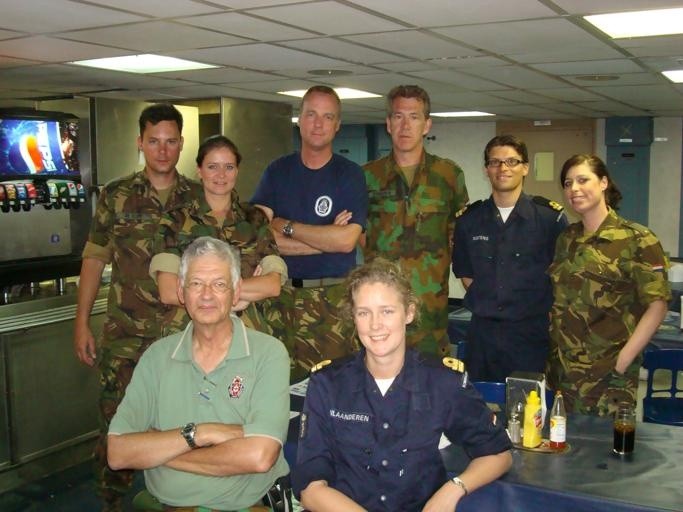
[180,423,201,451]
[282,218,296,237]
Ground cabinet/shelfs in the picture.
[0,286,109,491]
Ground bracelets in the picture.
[453,477,470,496]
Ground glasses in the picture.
[182,277,232,293]
[486,158,525,167]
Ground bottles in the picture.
[523,391,543,448]
[549,391,566,449]
[507,415,520,444]
[612,404,636,454]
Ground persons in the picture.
[249,85,368,386]
[292,259,513,512]
[149,133,288,339]
[546,151,672,417]
[106,235,290,512]
[359,83,473,359]
[451,132,572,384]
[74,99,205,512]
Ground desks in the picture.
[290,370,683,512]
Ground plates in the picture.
[510,438,572,455]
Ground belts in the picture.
[285,276,347,288]
